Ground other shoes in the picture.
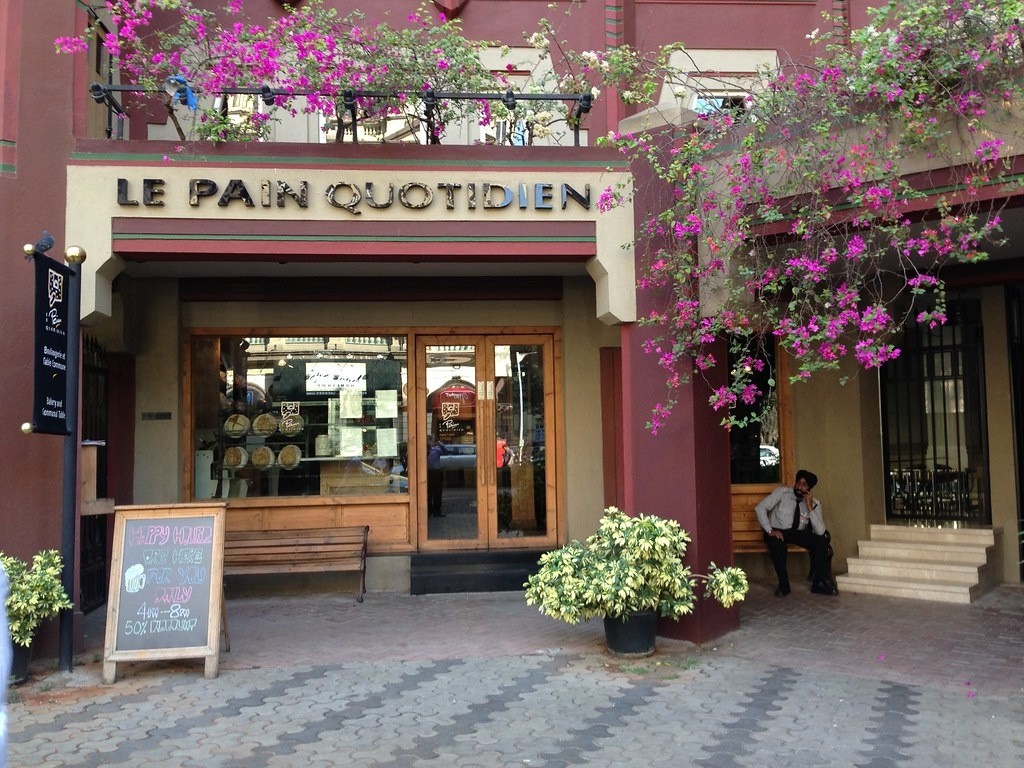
[775,581,791,597]
[810,581,838,596]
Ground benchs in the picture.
[223,525,371,602]
[729,482,810,553]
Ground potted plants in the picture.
[0,545,76,689]
[520,505,749,655]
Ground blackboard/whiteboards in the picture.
[104,502,226,662]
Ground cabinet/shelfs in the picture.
[210,408,402,499]
[327,395,378,454]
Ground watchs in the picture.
[768,530,773,534]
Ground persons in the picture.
[427,435,450,518]
[331,442,408,494]
[502,444,515,489]
[755,470,839,595]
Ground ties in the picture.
[790,499,803,530]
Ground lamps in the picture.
[287,353,292,359]
[342,89,356,109]
[377,354,384,358]
[91,83,106,104]
[424,89,437,110]
[346,354,353,359]
[317,352,323,357]
[503,89,518,110]
[580,91,594,113]
[178,89,188,106]
[261,84,276,105]
